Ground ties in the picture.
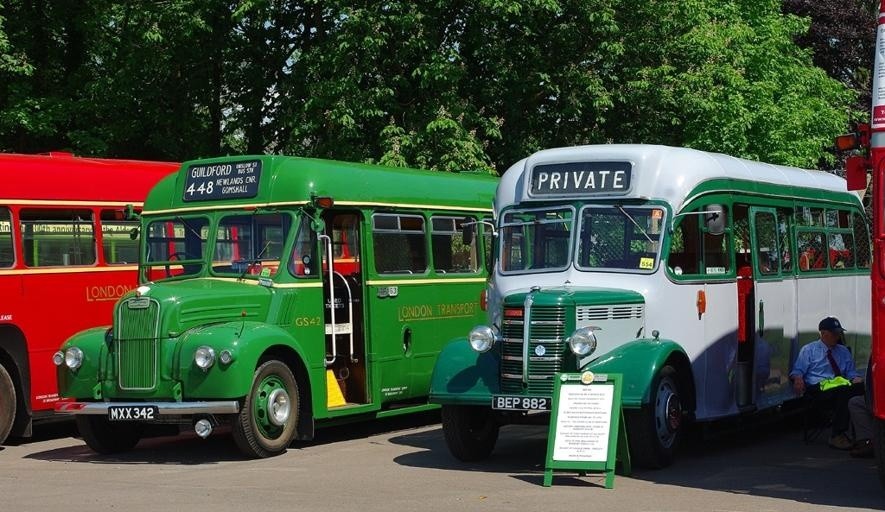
[827,349,841,378]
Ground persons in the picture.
[848,349,875,458]
[727,331,770,400]
[789,317,865,450]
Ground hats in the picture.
[819,317,847,335]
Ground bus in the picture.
[428,142,872,471]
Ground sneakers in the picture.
[849,441,874,457]
[830,432,853,450]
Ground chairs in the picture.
[790,338,856,445]
[738,246,870,342]
[324,270,478,352]
